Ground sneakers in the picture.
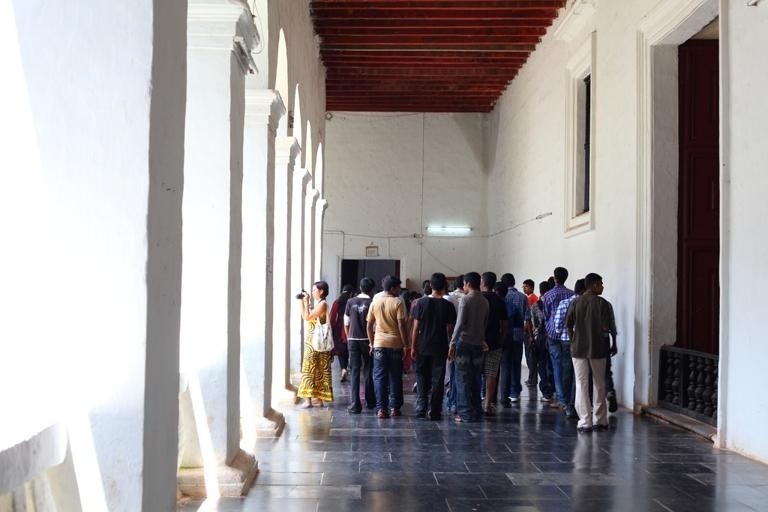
[541,397,551,401]
[511,398,518,402]
[579,428,593,432]
[377,412,470,422]
[593,425,608,429]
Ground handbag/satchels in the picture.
[312,323,335,352]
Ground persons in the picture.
[297,281,334,408]
[522,268,619,432]
[330,272,528,423]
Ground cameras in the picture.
[296,289,306,299]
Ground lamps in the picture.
[423,225,472,233]
